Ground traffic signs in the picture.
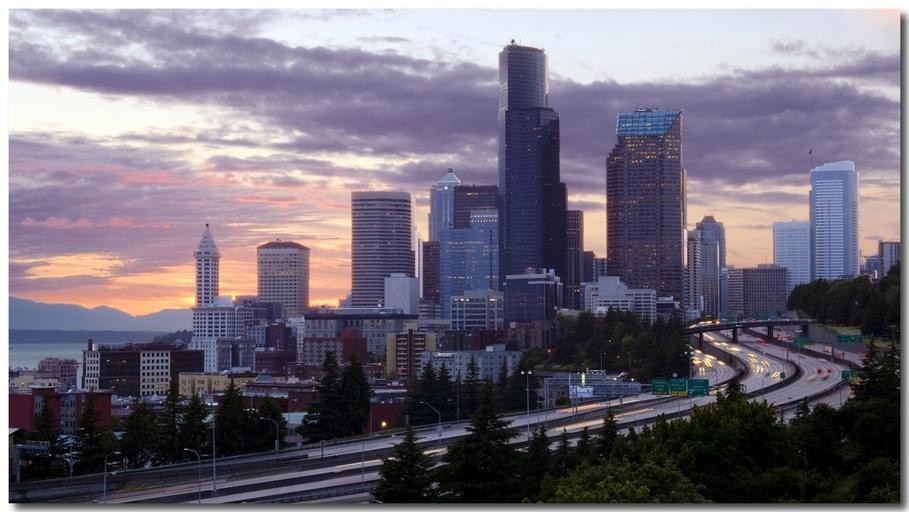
[687,379,709,396]
[841,371,851,382]
[668,378,689,397]
[838,335,864,343]
[651,378,669,396]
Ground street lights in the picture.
[520,368,533,440]
[184,448,202,505]
[418,401,442,448]
[101,450,122,505]
[259,416,279,454]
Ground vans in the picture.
[589,369,606,376]
